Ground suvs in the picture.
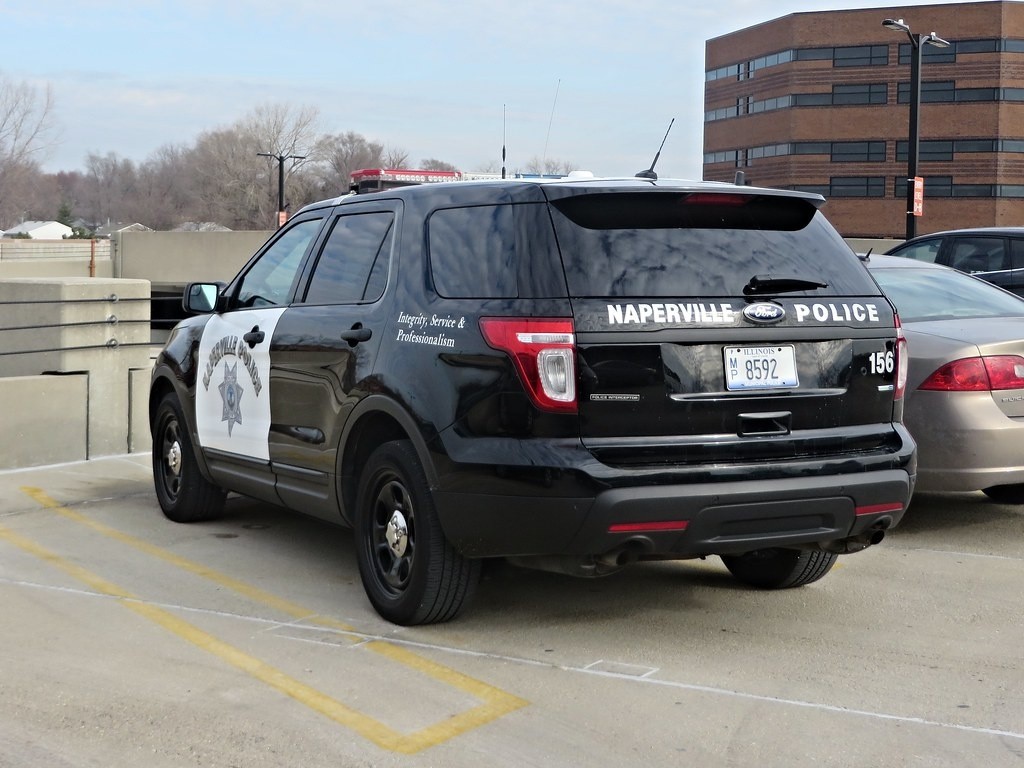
[145,177,917,632]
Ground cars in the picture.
[856,251,1024,503]
[880,229,1024,294]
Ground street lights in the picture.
[255,152,306,229]
[882,16,955,244]
